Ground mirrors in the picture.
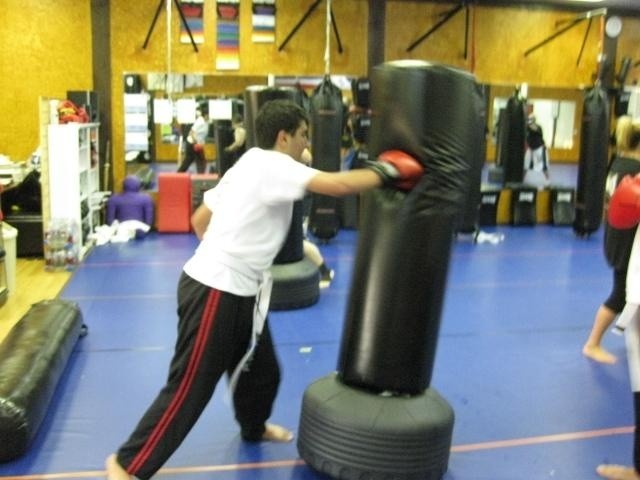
[124,70,583,196]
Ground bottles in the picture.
[41,216,79,272]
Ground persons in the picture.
[581,114,640,365]
[596,226,640,479]
[223,113,245,165]
[300,148,335,289]
[106,174,154,239]
[524,112,553,184]
[104,98,424,479]
[177,107,210,174]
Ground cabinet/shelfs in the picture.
[49,120,104,265]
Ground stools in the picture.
[5,211,44,258]
[0,221,19,294]
